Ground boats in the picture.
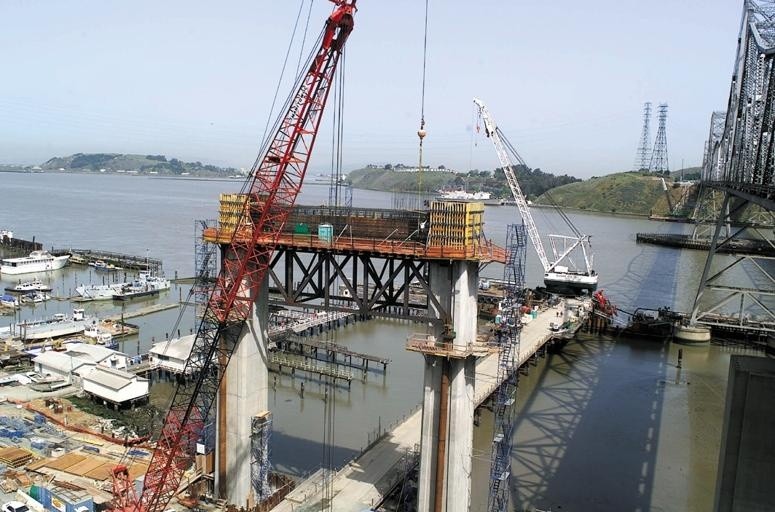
[434,188,504,207]
[110,249,171,298]
[69,255,123,272]
[0,248,70,274]
[0,276,55,311]
[26,371,43,383]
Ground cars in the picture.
[1,500,33,512]
